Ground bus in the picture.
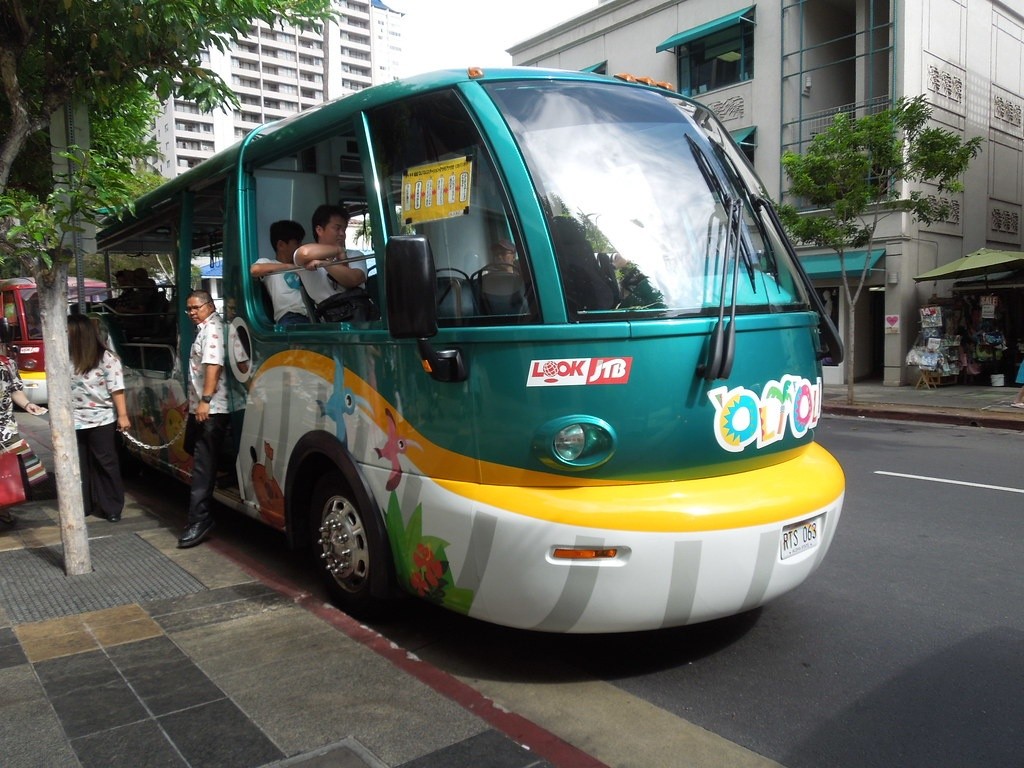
[0,277,113,404]
[68,67,846,634]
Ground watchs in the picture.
[202,395,212,403]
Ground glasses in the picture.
[184,301,210,314]
[493,251,514,257]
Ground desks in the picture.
[908,363,938,390]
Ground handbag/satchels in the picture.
[318,286,381,320]
[0,441,48,508]
[906,334,927,366]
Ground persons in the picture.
[179,290,232,550]
[489,239,516,274]
[293,204,381,323]
[0,352,49,534]
[248,220,319,326]
[218,295,253,441]
[1010,340,1024,408]
[26,312,44,339]
[99,266,200,350]
[65,314,132,523]
[4,292,17,325]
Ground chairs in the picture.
[436,262,530,326]
[299,277,324,324]
[121,284,169,344]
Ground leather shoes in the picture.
[179,514,216,546]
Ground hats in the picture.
[491,239,515,252]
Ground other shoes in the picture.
[108,512,120,522]
[1011,400,1024,408]
